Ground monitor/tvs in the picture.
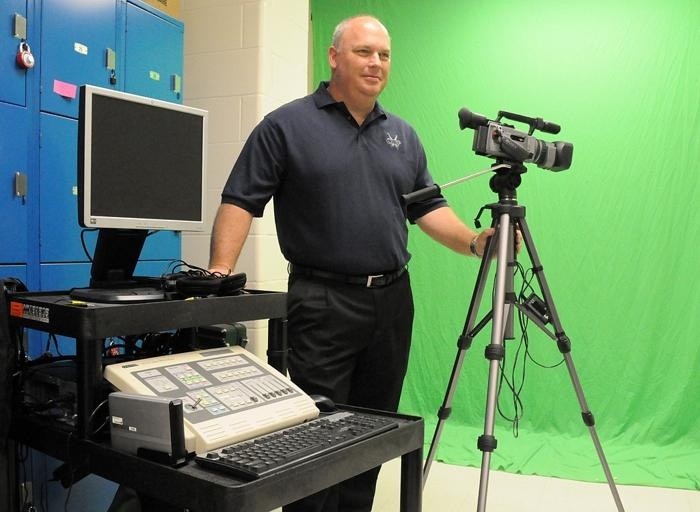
[69,83,210,304]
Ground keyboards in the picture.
[193,409,399,482]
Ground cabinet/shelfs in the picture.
[1,273,424,512]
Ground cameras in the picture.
[457,106,574,173]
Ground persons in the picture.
[206,15,522,512]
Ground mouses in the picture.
[309,394,337,413]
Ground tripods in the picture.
[422,173,626,512]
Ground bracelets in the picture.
[470,235,483,259]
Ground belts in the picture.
[290,262,405,289]
